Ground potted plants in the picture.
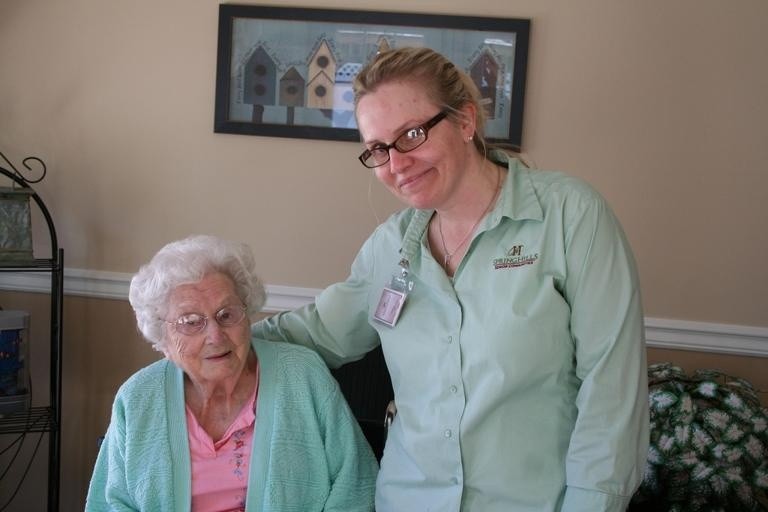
[626,363,768,511]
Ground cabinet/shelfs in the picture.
[0,144,72,512]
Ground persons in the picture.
[249,46,654,512]
[83,233,379,510]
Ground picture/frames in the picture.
[210,1,534,157]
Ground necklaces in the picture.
[438,159,500,272]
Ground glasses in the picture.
[358,96,466,169]
[159,303,248,336]
